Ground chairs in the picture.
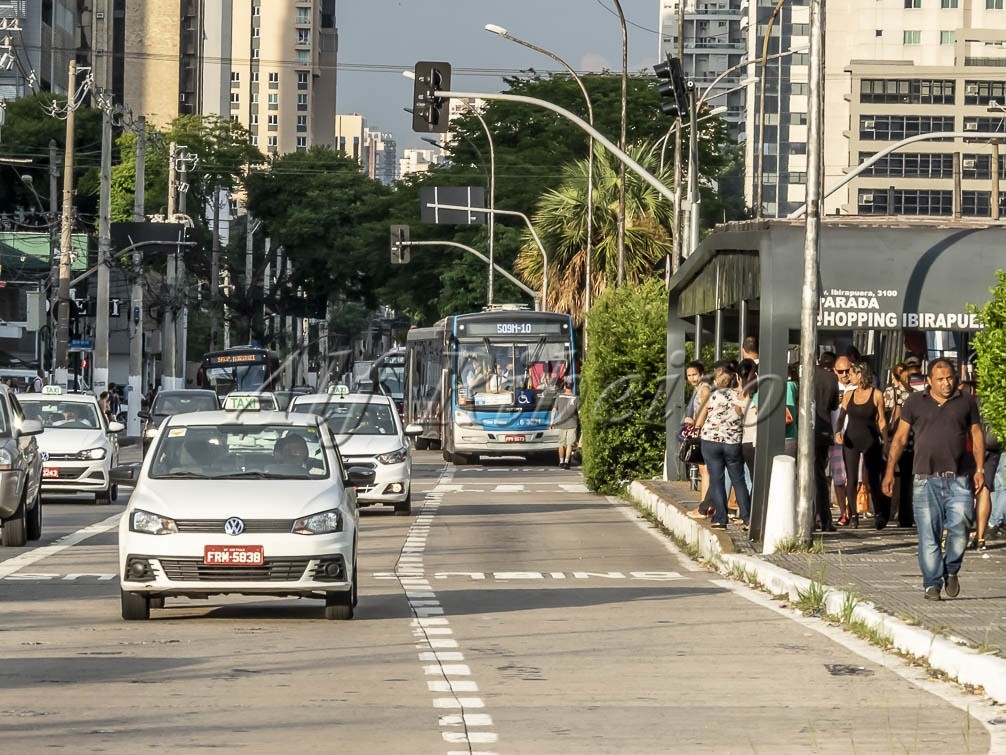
[273,434,309,469]
[181,441,213,472]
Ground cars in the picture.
[283,384,424,513]
[218,391,315,460]
[14,384,123,500]
[119,392,376,622]
[0,384,48,547]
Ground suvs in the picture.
[140,386,229,462]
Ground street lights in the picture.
[645,47,816,368]
[21,173,58,373]
[484,25,590,384]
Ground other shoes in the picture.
[836,518,848,526]
[563,463,570,469]
[944,572,959,596]
[560,462,563,468]
[924,587,941,601]
[970,537,986,551]
[849,517,860,529]
[742,525,749,531]
[710,523,726,530]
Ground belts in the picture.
[932,470,968,479]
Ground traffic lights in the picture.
[654,55,690,124]
[67,351,84,376]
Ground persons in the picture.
[108,382,121,415]
[816,345,919,532]
[958,380,1006,550]
[904,352,929,392]
[98,391,109,414]
[33,369,44,392]
[460,347,523,405]
[882,357,985,602]
[549,380,579,470]
[3,376,18,390]
[551,365,564,389]
[53,404,92,426]
[742,336,759,374]
[265,434,324,474]
[23,405,44,424]
[686,358,797,531]
[349,407,386,433]
[536,373,559,390]
[146,384,158,409]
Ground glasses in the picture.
[835,370,850,374]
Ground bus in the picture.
[195,345,290,396]
[347,346,406,413]
[401,303,582,465]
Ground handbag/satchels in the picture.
[678,428,705,464]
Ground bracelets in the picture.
[976,468,984,473]
